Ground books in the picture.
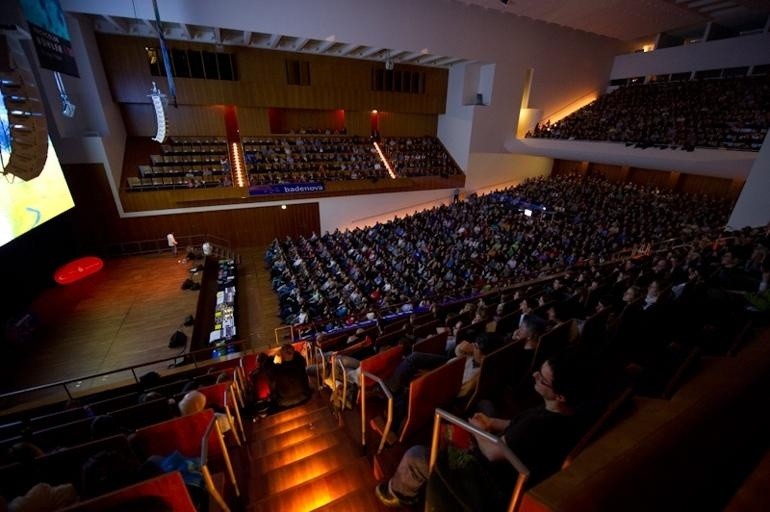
[209,258,237,358]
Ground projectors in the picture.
[62,102,76,119]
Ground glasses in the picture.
[539,373,551,388]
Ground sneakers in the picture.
[376,481,417,507]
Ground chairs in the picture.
[126,136,228,191]
[1,355,249,510]
[313,268,767,509]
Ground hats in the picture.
[178,390,206,415]
[8,482,75,511]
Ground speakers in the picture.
[182,278,194,289]
[168,330,187,348]
[186,252,195,259]
[184,314,193,326]
[193,264,204,274]
[192,282,201,291]
[196,252,203,260]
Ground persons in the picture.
[0,364,237,512]
[183,123,461,188]
[247,352,275,406]
[202,239,214,258]
[523,72,769,153]
[265,166,769,510]
[166,230,179,256]
